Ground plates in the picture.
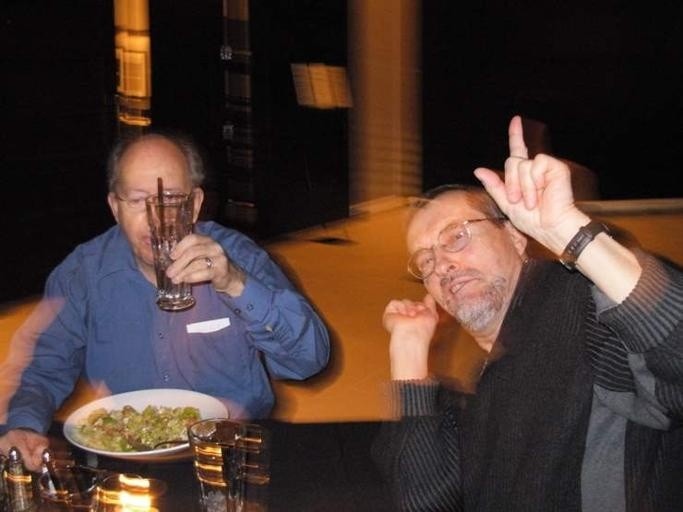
[62,388,230,456]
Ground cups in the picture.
[96,471,169,512]
[37,466,100,511]
[146,195,198,310]
[187,417,247,511]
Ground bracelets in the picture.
[555,220,610,269]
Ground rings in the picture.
[203,255,214,270]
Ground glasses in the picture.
[408,218,506,279]
[114,188,196,210]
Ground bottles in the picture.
[0,444,33,512]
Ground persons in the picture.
[380,114,680,507]
[1,130,329,472]
